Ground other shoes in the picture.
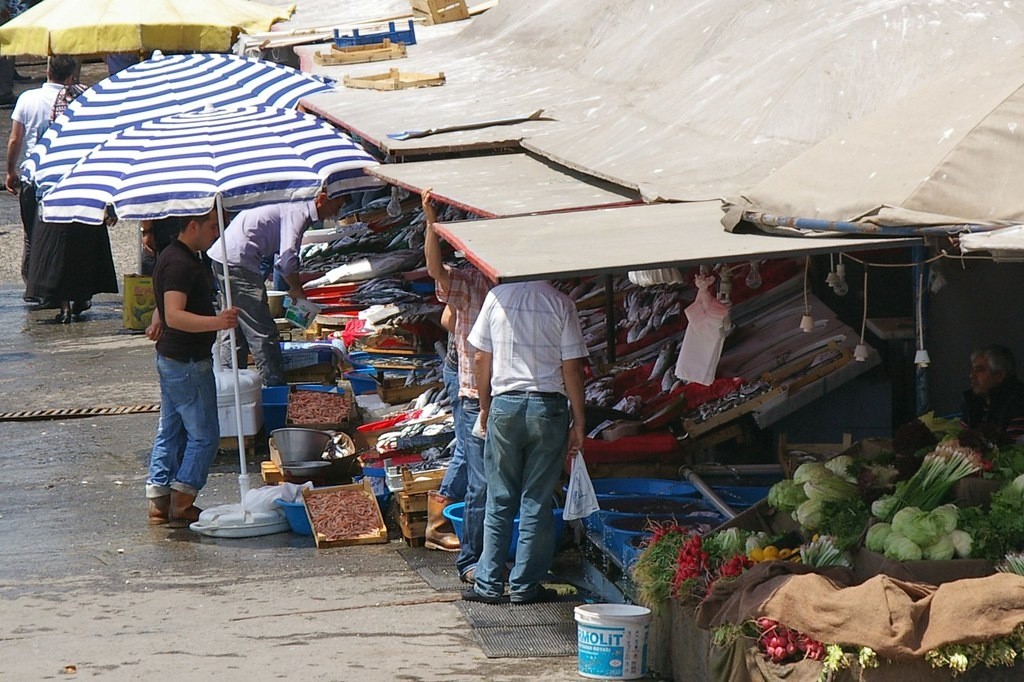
[463,568,476,583]
[71,300,92,315]
[55,312,70,323]
[461,587,500,604]
[514,584,558,603]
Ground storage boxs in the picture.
[218,398,263,438]
[261,385,290,438]
[123,274,157,331]
[397,466,448,548]
[377,378,445,402]
[302,478,389,548]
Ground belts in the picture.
[503,389,562,398]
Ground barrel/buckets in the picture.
[574,603,653,680]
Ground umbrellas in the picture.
[19,48,338,197]
[37,102,389,524]
[0,0,297,55]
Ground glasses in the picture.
[970,364,990,372]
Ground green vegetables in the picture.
[818,450,923,550]
[935,428,1024,560]
[822,621,1024,677]
[705,526,786,557]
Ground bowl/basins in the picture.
[563,477,773,571]
[267,291,289,319]
[270,428,332,463]
[442,503,567,561]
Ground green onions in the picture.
[884,440,983,521]
[992,551,1024,576]
[799,535,854,570]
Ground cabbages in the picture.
[767,454,860,530]
[865,497,974,562]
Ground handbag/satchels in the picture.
[562,451,599,520]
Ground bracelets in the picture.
[140,226,152,234]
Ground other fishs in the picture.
[298,196,478,475]
[568,278,684,422]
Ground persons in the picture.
[420,188,496,584]
[23,84,119,323]
[961,344,1024,452]
[205,187,353,387]
[5,54,78,311]
[140,216,179,276]
[460,279,590,604]
[424,259,477,551]
[145,202,240,526]
[0,0,44,109]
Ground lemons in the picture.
[750,535,818,563]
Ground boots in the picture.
[146,483,170,525]
[424,489,460,552]
[165,482,204,528]
